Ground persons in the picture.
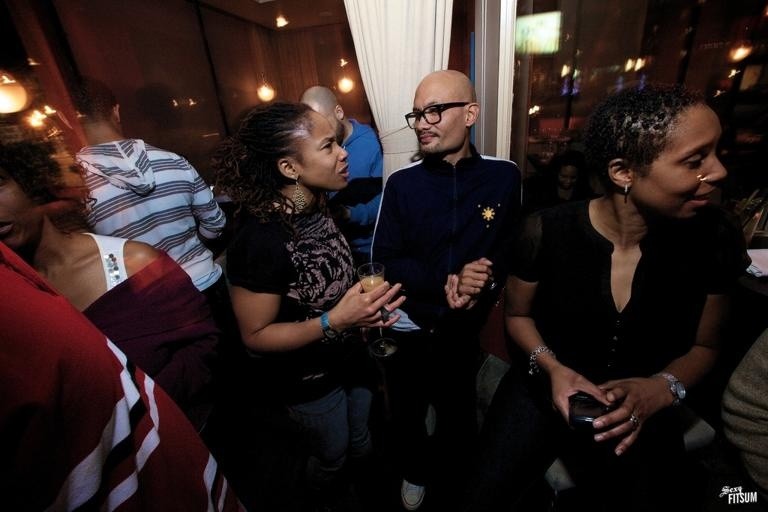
[1,241,246,512]
[538,149,586,205]
[503,84,751,512]
[219,102,406,512]
[69,75,244,362]
[1,122,222,447]
[301,86,384,266]
[370,70,524,510]
[720,330,768,494]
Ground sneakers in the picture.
[400,476,428,511]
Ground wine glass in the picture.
[358,262,399,358]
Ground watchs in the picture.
[652,372,687,406]
[320,312,339,341]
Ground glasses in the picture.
[403,102,474,130]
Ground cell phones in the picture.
[569,394,607,427]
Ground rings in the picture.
[379,306,389,323]
[628,413,639,431]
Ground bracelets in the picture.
[527,345,556,375]
